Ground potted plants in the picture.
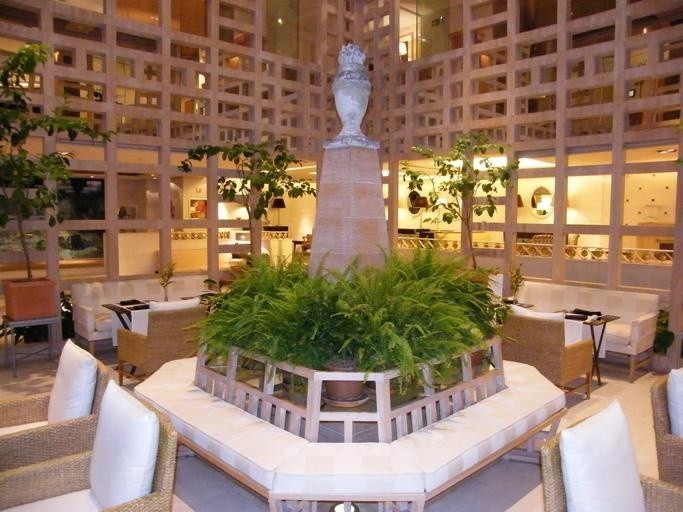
[267,266,423,407]
[153,261,179,302]
[0,43,115,320]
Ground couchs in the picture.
[71,276,209,386]
[490,270,661,399]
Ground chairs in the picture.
[539,407,682,511]
[0,338,110,436]
[649,363,682,487]
[0,385,177,511]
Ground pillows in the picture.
[666,368,683,432]
[559,399,645,511]
[90,379,160,506]
[46,338,97,424]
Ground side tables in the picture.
[1,316,64,377]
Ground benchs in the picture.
[131,357,564,512]
